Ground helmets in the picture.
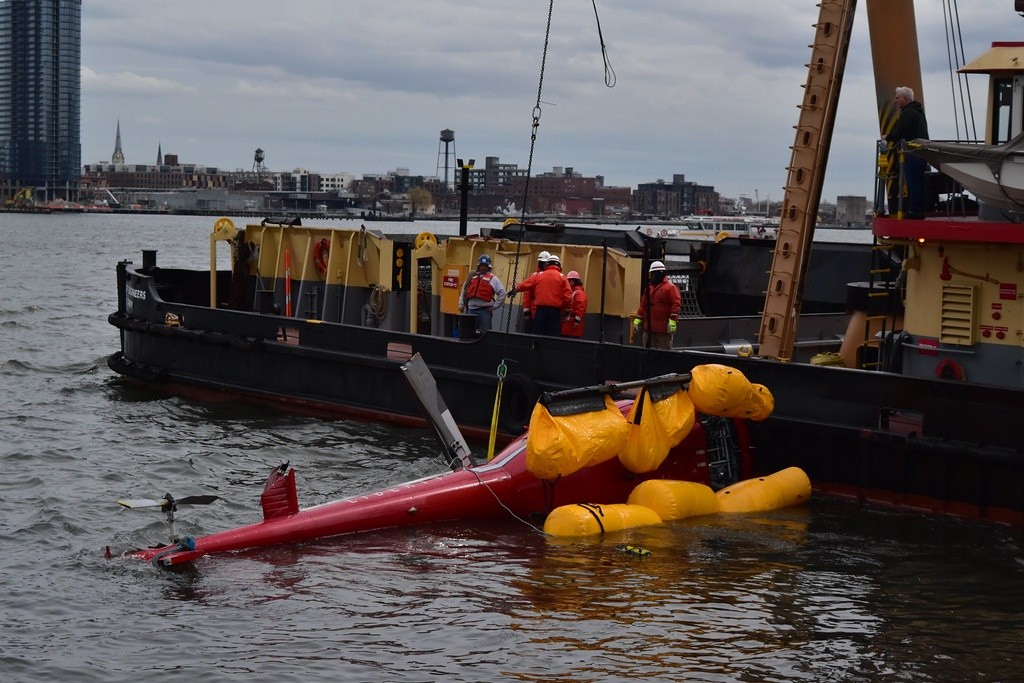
[537,251,551,261]
[648,261,666,272]
[567,271,580,279]
[547,255,560,262]
[478,254,491,264]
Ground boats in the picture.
[360,211,414,221]
[105,0,1024,537]
[905,136,1024,217]
[656,213,782,241]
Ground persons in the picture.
[506,251,587,339]
[633,261,681,350]
[457,253,506,331]
[882,86,929,220]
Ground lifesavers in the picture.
[661,230,667,236]
[312,238,331,278]
[647,229,652,235]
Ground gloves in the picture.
[458,307,464,313]
[574,316,580,326]
[633,319,642,334]
[666,319,677,334]
[506,288,518,298]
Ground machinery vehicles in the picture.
[5,187,32,209]
